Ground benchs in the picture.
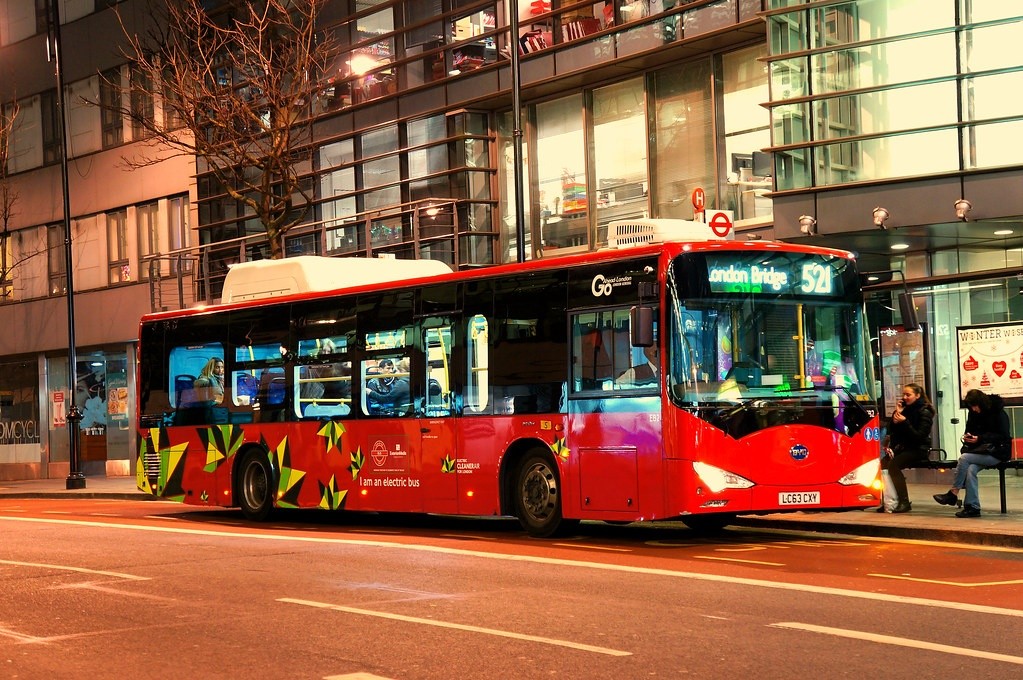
[910,449,1023,514]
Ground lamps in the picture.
[954,199,973,223]
[799,214,818,235]
[872,206,890,229]
[747,233,762,241]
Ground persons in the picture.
[196,357,243,406]
[881,383,936,513]
[613,340,657,386]
[366,357,410,415]
[301,338,350,398]
[77,363,106,414]
[933,389,1012,517]
[367,359,400,415]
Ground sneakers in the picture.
[933,492,958,506]
[955,504,982,519]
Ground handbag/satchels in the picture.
[881,468,899,513]
[960,435,995,455]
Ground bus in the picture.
[135,217,919,537]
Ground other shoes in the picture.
[877,506,885,513]
[891,500,912,513]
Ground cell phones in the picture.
[966,436,973,440]
[901,399,906,407]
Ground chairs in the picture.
[236,371,286,405]
[175,374,196,407]
